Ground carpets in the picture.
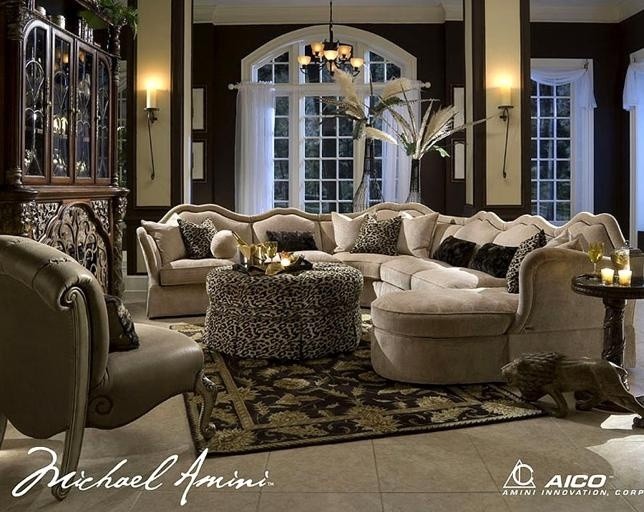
[170,319,552,459]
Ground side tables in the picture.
[570,273,644,412]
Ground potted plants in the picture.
[317,69,430,212]
[365,83,505,207]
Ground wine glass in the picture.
[588,241,604,276]
[265,241,278,261]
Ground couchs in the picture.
[135,204,638,385]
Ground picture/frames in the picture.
[192,85,207,133]
[450,82,465,183]
[191,140,206,183]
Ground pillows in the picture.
[432,235,475,268]
[506,230,546,292]
[349,215,402,256]
[104,294,140,353]
[558,239,583,254]
[267,232,318,252]
[469,243,517,278]
[331,211,373,252]
[397,211,438,259]
[177,218,218,259]
[543,229,574,248]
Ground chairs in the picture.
[0,232,208,502]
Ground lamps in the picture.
[296,1,363,79]
[496,73,514,176]
[144,79,160,177]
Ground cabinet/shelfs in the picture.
[83,1,139,55]
[0,5,126,307]
